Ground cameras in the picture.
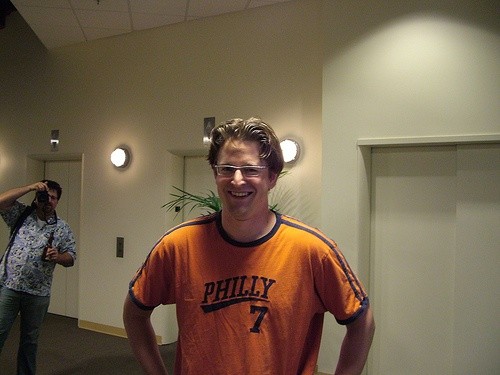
[37,191,48,202]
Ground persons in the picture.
[122,117,375,375]
[0,179,77,375]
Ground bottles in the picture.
[40,231,54,262]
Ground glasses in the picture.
[214,164,270,177]
